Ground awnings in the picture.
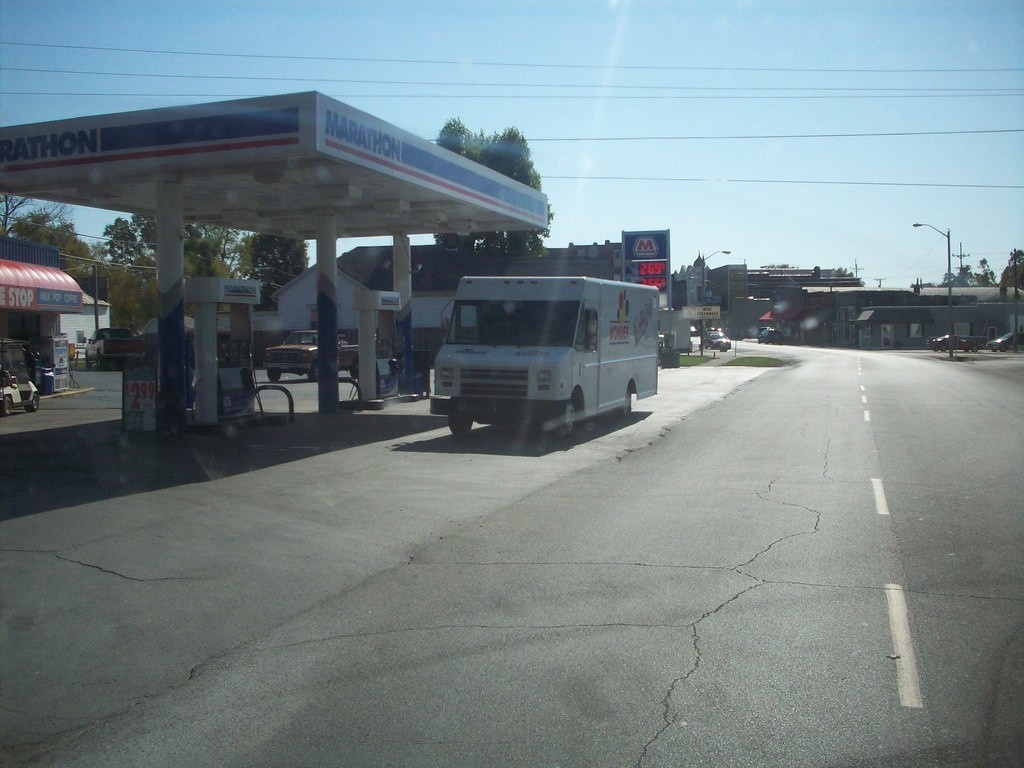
[0,258,84,314]
[758,309,805,321]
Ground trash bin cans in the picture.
[661,351,681,368]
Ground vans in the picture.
[987,332,1024,352]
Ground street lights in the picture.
[701,250,732,356]
[912,223,954,358]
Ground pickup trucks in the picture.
[262,330,359,382]
[81,326,146,371]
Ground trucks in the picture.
[926,334,987,353]
[429,275,661,440]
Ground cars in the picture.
[701,331,731,352]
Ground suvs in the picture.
[757,328,784,345]
